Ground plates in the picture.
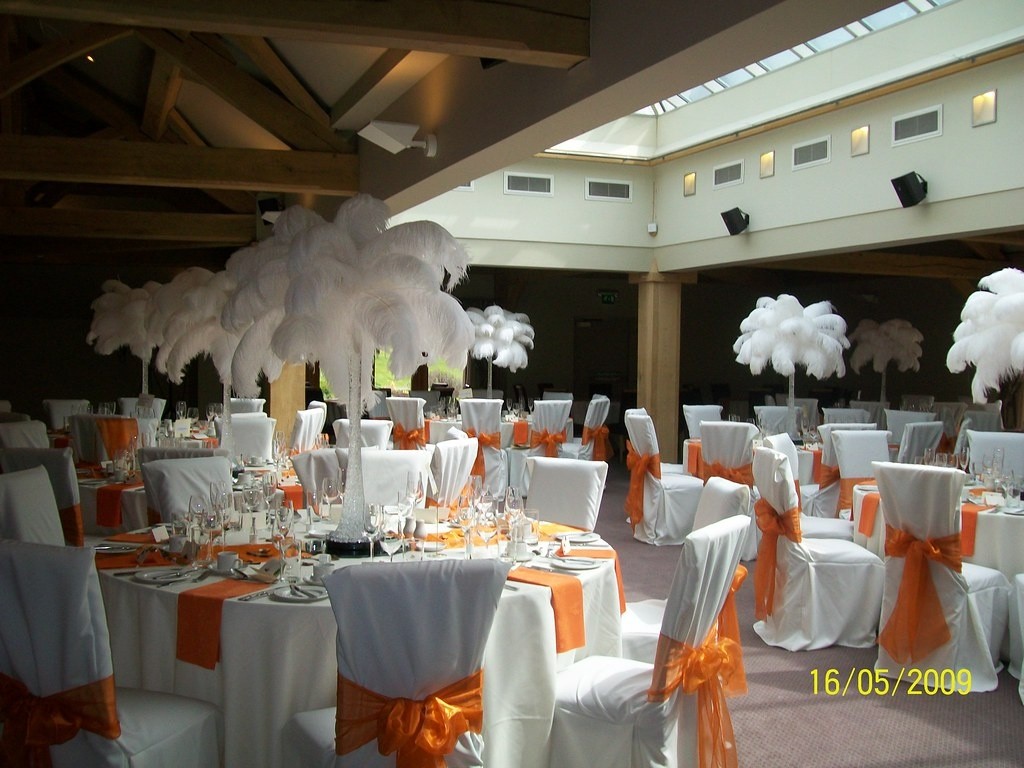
[94,544,137,554]
[304,572,330,584]
[208,562,247,573]
[418,541,446,551]
[135,569,189,582]
[552,556,601,570]
[556,531,601,543]
[273,586,329,602]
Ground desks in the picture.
[42,419,1024,768]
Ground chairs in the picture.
[0,396,1024,768]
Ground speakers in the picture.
[720,207,746,236]
[891,171,925,209]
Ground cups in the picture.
[914,446,971,474]
[70,400,116,415]
[312,563,334,581]
[110,426,173,479]
[281,539,302,583]
[246,457,265,465]
[217,550,244,573]
[728,414,755,425]
[307,487,323,524]
[404,538,423,562]
[498,509,540,563]
[434,396,460,421]
[166,518,189,550]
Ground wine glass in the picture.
[176,402,222,438]
[457,475,529,560]
[322,468,347,521]
[189,472,294,562]
[800,417,817,451]
[980,448,1021,510]
[506,396,542,422]
[362,469,427,563]
[272,430,331,474]
